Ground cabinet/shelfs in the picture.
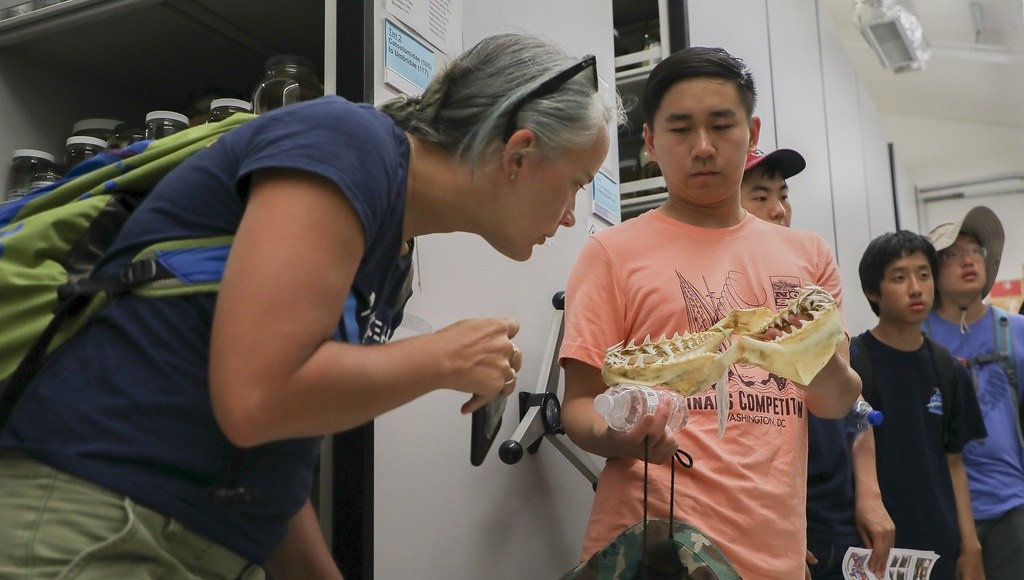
[0,1,369,218]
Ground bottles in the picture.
[844,400,884,433]
[249,54,323,116]
[6,109,191,199]
[612,19,668,196]
[593,383,690,434]
[203,97,254,124]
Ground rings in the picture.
[510,344,517,363]
[505,369,516,385]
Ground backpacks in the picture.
[0,112,416,431]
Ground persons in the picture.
[1,34,609,580]
[739,148,1024,580]
[557,44,863,580]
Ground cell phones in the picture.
[471,393,503,467]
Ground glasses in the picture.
[502,54,599,144]
[938,246,988,263]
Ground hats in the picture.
[927,204,1005,299]
[560,518,743,580]
[744,147,806,179]
